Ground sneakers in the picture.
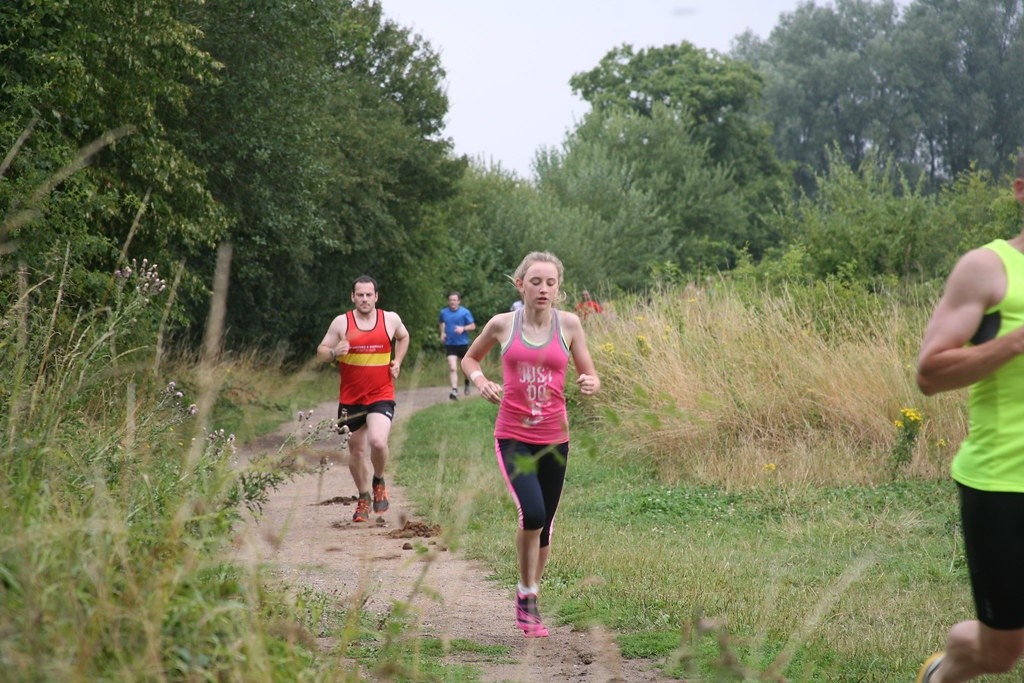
[370,479,390,515]
[523,626,550,638]
[352,498,373,522]
[513,585,544,632]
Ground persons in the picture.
[461,252,605,637]
[919,150,1024,683]
[317,275,409,522]
[439,291,476,399]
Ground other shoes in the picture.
[450,391,459,400]
[463,383,470,396]
[913,650,947,683]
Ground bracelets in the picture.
[331,348,336,360]
